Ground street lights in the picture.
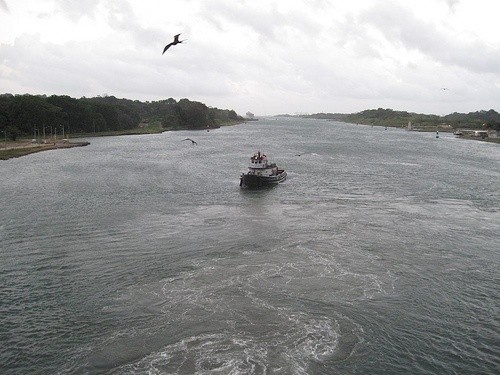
[1,129,7,150]
[32,124,64,146]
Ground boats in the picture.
[238,151,287,190]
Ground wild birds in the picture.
[162,34,189,54]
[183,138,198,146]
[440,88,448,90]
[294,153,308,157]
